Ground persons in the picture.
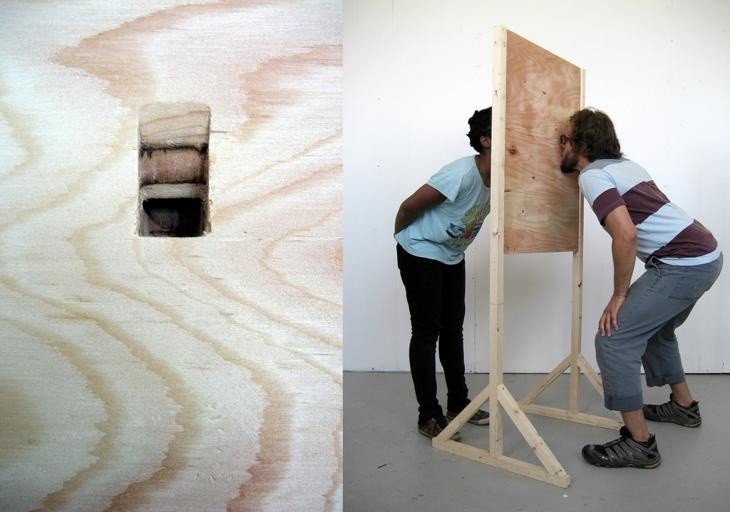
[559,108,724,468]
[391,107,489,439]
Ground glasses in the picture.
[560,135,575,145]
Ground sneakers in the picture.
[418,415,461,443]
[582,424,661,469]
[643,392,702,427]
[446,402,489,426]
